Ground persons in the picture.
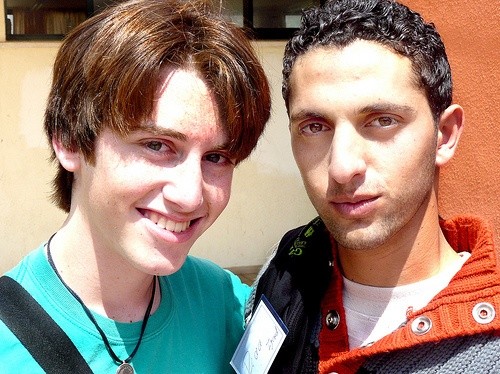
[1,0,274,374]
[245,0,500,374]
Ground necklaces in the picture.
[47,231,159,374]
